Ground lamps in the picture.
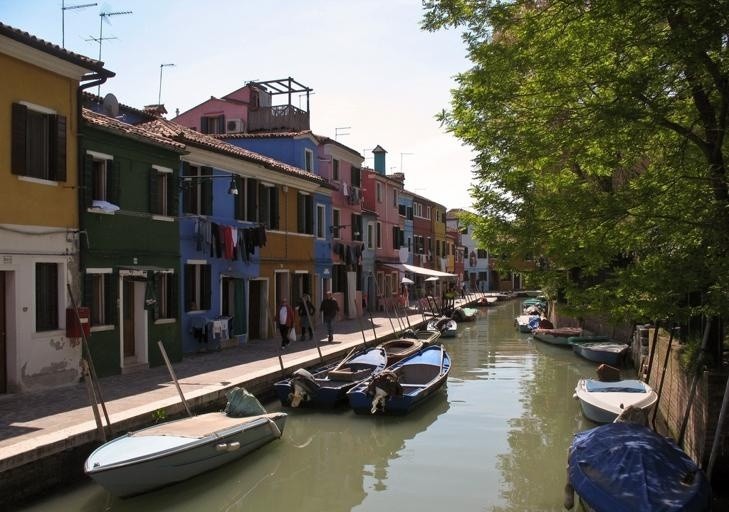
[177,171,240,196]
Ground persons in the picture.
[276,291,340,349]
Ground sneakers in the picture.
[280,339,293,350]
[300,335,333,341]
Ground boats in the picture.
[515,296,583,346]
[83,387,289,500]
[345,344,453,417]
[572,363,658,426]
[273,347,387,408]
[567,335,629,366]
[400,330,442,347]
[564,405,705,512]
[478,297,498,306]
[426,315,458,337]
[375,328,424,366]
[451,306,478,321]
